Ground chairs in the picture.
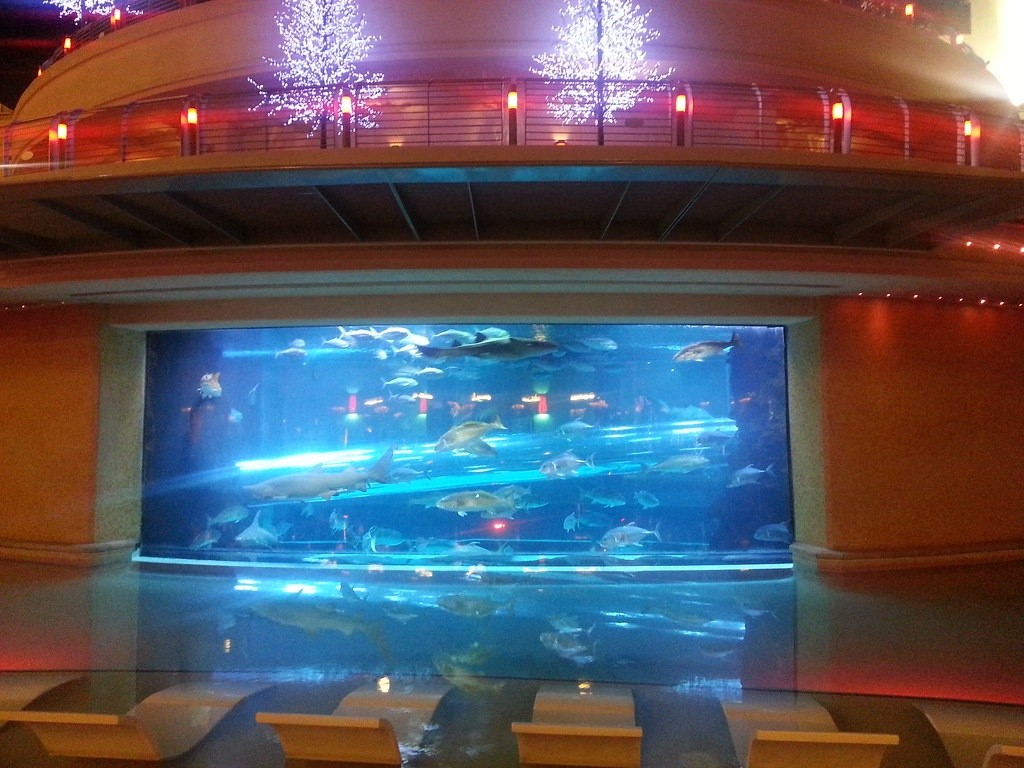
[0,668,1024,768]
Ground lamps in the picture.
[506,83,520,109]
[340,88,351,115]
[186,102,199,124]
[964,114,970,136]
[675,87,688,113]
[57,116,68,141]
[832,95,844,120]
[555,139,566,146]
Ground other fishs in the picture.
[181,325,794,692]
[434,596,518,618]
[243,442,398,501]
